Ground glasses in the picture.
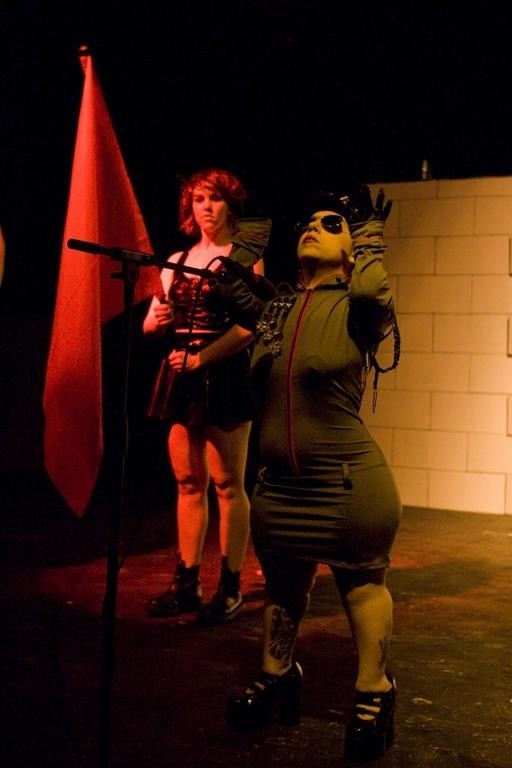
[295,215,341,231]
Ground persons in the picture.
[141,165,274,628]
[211,172,401,764]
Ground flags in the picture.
[41,53,166,518]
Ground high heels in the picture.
[226,662,303,728]
[344,669,397,759]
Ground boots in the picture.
[145,551,202,616]
[198,555,244,625]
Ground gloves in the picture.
[344,185,392,225]
[230,190,271,219]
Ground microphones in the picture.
[218,255,279,302]
